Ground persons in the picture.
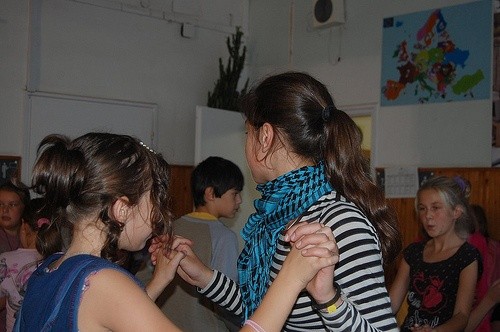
[0,179,31,255]
[388,175,483,332]
[0,198,62,332]
[458,205,500,332]
[149,72,401,332]
[11,133,339,332]
[155,157,244,332]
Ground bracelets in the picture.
[311,281,341,309]
[243,320,266,332]
